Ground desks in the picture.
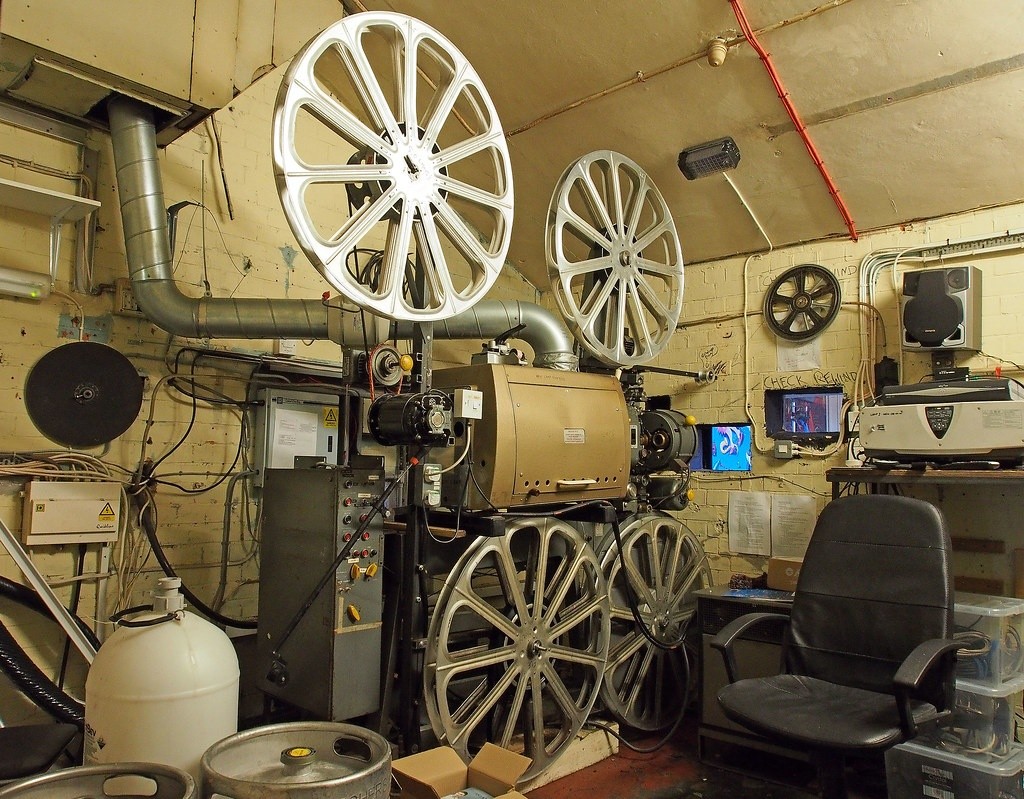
[825,466,1024,501]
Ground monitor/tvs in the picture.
[688,428,712,471]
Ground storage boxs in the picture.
[908,673,1024,762]
[391,742,533,799]
[884,727,1024,799]
[954,591,1024,690]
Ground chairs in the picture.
[715,494,970,799]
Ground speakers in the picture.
[900,266,982,351]
